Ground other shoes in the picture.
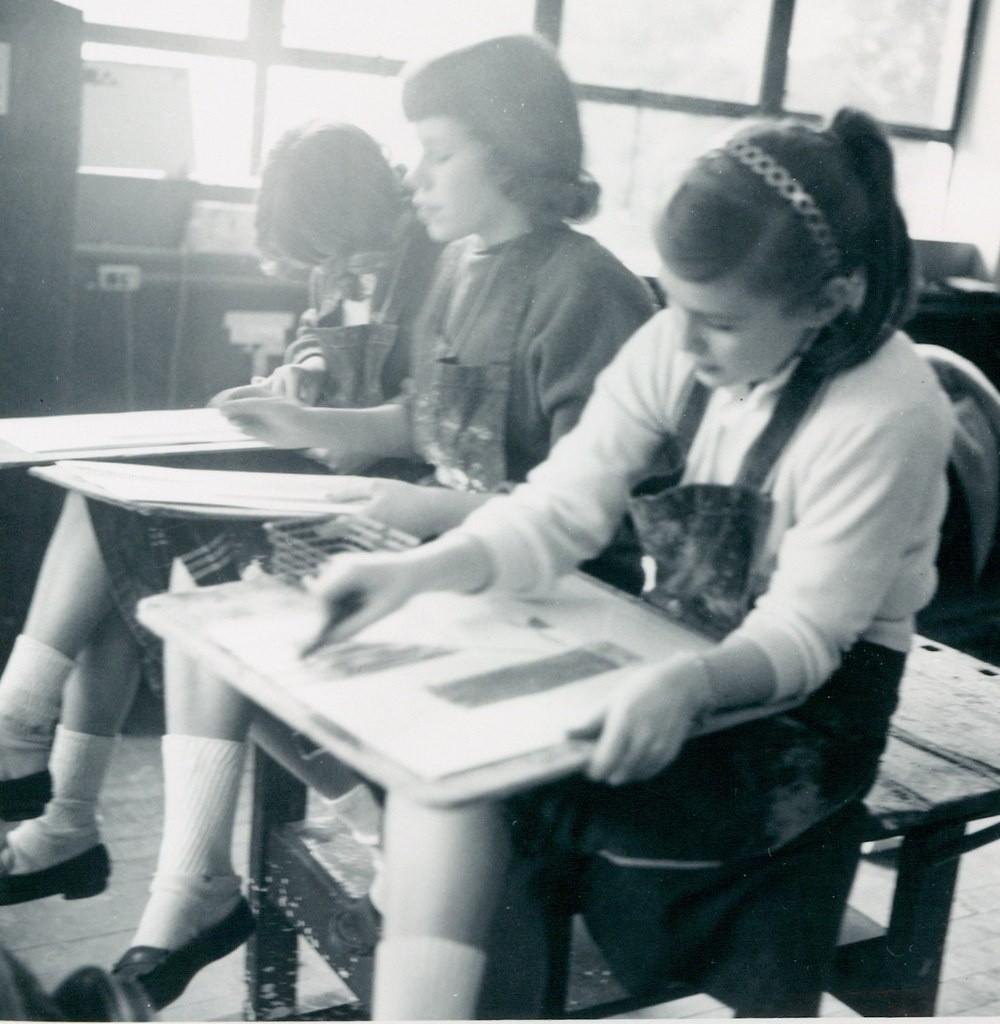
[0,769,55,821]
[0,842,111,907]
[110,895,257,1010]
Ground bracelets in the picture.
[694,654,718,721]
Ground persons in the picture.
[317,102,953,1021]
[111,31,665,1012]
[1,121,438,905]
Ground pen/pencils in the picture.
[297,603,358,663]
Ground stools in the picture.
[239,627,1000,1017]
